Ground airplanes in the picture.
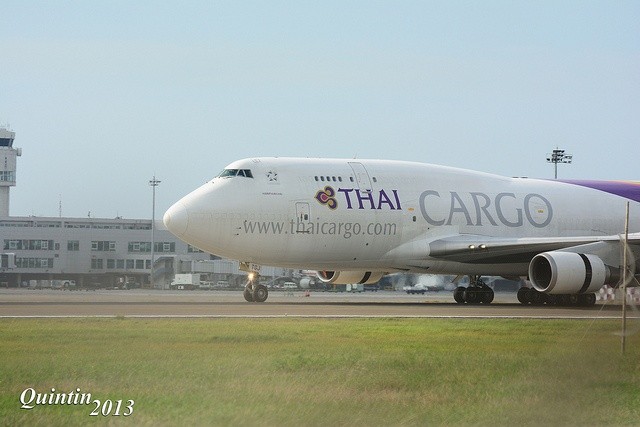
[163,155,637,307]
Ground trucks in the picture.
[170,273,200,289]
[403,283,429,295]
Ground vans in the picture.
[283,282,298,289]
[217,280,230,288]
[202,281,214,291]
[200,280,204,288]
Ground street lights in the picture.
[148,176,161,288]
